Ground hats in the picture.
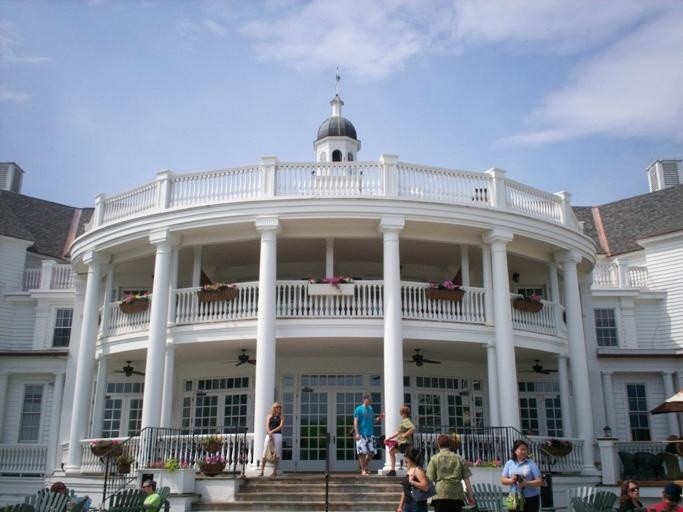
[663,482,682,495]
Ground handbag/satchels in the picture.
[505,492,525,511]
[265,439,280,465]
[410,480,437,501]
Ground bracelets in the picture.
[376,417,380,422]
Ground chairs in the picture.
[107,486,171,512]
[462,482,509,512]
[0,487,91,511]
[541,485,618,512]
[617,450,683,480]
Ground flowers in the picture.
[544,439,572,449]
[89,439,119,448]
[518,294,542,306]
[467,457,504,469]
[206,456,226,464]
[430,279,460,291]
[117,295,150,305]
[143,456,189,472]
[200,280,237,293]
[309,274,353,293]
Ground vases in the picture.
[136,469,196,495]
[308,283,355,296]
[541,446,572,456]
[425,287,466,300]
[197,289,236,302]
[513,298,543,312]
[462,466,508,492]
[199,464,225,476]
[91,447,123,456]
[119,301,148,313]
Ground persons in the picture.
[142,480,161,512]
[646,483,683,512]
[397,446,429,512]
[354,394,384,476]
[50,482,67,512]
[502,440,543,512]
[620,480,642,512]
[385,406,416,476]
[259,403,284,477]
[665,435,683,474]
[426,435,475,512]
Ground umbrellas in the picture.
[650,391,683,415]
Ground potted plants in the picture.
[115,446,136,474]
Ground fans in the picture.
[112,360,145,377]
[402,347,441,368]
[222,348,255,367]
[517,359,558,375]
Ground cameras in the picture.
[515,474,523,485]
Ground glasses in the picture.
[628,487,639,492]
